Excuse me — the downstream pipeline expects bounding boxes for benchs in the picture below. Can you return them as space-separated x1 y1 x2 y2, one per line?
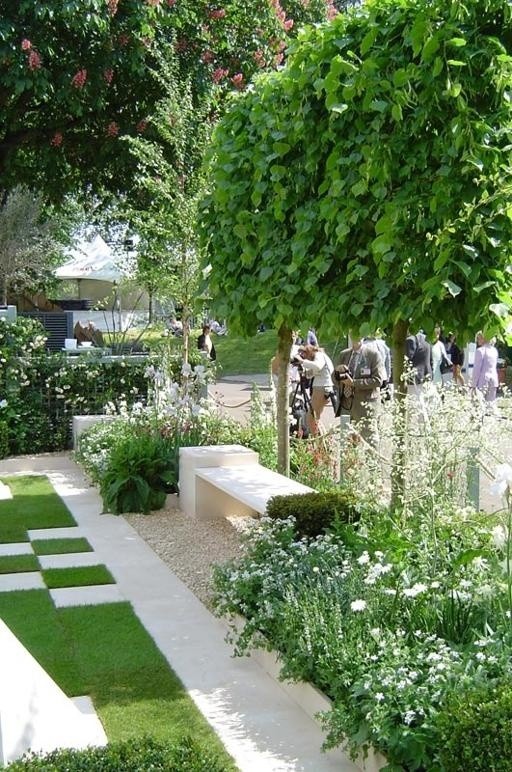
178 443 318 518
63 347 112 356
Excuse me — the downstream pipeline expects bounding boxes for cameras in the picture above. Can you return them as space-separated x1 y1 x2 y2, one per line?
290 357 303 368
335 366 352 381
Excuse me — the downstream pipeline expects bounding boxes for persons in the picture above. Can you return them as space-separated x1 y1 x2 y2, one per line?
161 315 216 363
271 319 499 477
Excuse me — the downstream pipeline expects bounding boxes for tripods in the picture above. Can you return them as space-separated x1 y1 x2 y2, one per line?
289 369 315 438
